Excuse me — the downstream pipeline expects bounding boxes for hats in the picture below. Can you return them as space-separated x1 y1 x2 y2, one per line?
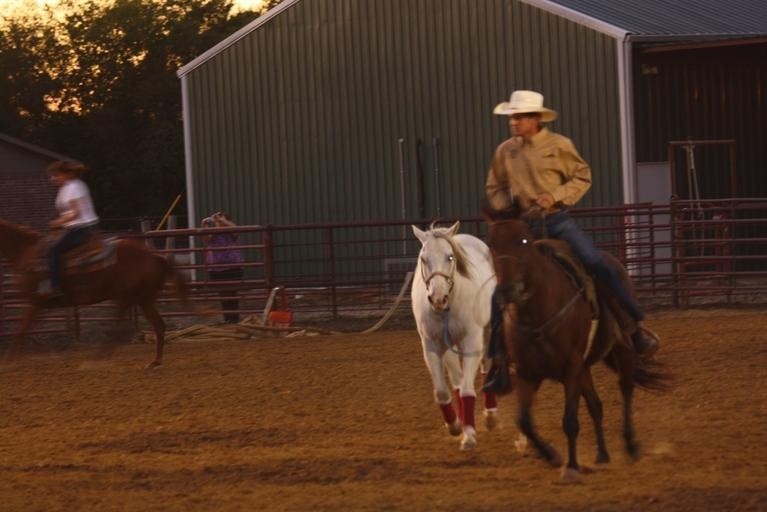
493 90 557 123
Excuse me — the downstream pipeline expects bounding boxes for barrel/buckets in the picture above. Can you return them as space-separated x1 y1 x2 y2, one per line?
268 312 292 335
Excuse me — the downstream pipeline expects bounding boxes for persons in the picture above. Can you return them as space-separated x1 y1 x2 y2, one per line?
481 86 679 399
200 212 246 329
34 156 103 301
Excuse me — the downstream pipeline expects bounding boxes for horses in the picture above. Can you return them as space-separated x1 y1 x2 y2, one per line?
0 219 197 371
410 220 498 457
479 194 674 482
